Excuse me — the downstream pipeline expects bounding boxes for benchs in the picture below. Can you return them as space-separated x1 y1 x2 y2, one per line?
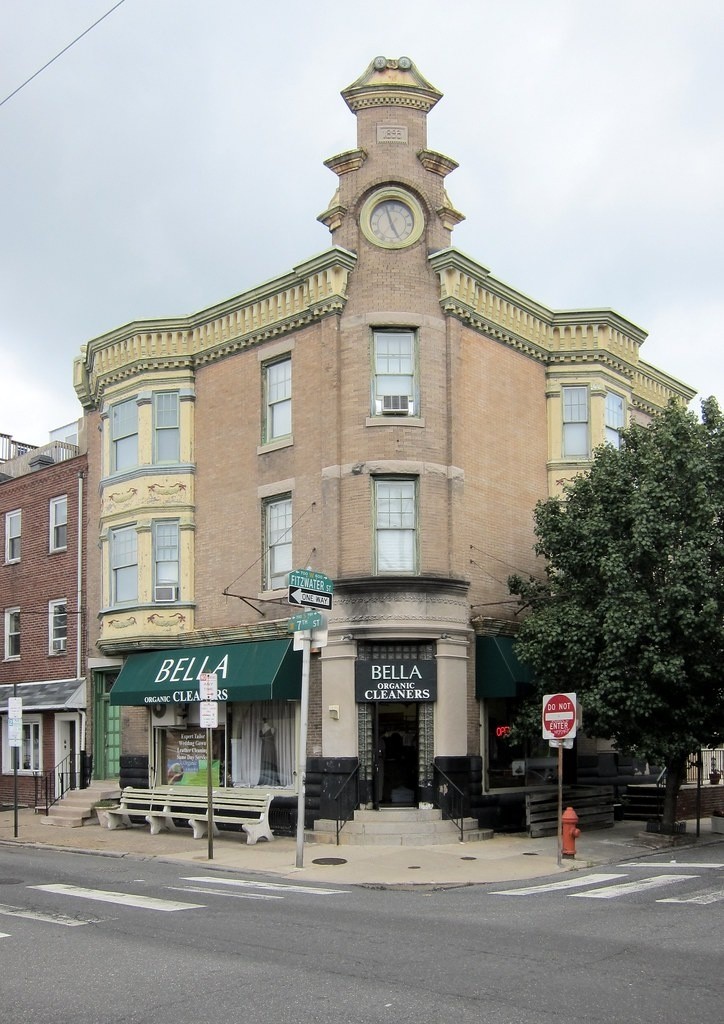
107 786 274 845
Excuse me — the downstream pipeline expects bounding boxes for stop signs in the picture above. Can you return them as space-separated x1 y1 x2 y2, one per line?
542 693 577 739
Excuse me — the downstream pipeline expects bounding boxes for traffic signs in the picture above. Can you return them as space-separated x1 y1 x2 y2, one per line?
287 585 333 612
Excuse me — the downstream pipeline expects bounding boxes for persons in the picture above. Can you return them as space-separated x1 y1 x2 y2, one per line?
257 717 280 786
167 763 184 785
377 727 387 804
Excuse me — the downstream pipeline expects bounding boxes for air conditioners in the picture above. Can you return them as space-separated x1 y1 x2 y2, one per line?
53 639 67 650
151 704 187 729
381 395 408 415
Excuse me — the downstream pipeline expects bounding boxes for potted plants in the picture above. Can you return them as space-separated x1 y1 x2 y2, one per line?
94 801 121 828
709 762 720 785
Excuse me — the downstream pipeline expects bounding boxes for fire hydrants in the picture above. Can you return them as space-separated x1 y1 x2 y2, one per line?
561 807 581 858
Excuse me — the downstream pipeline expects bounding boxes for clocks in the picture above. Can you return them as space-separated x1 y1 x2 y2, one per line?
358 187 425 249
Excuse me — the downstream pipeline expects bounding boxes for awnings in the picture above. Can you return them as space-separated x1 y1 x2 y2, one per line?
475 634 538 700
109 638 304 707
